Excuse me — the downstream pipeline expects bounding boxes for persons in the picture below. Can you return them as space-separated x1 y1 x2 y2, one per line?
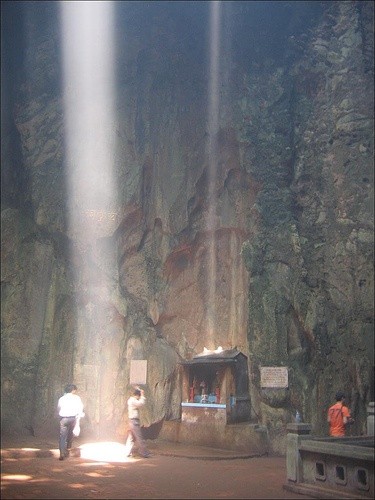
57 384 85 461
326 393 354 440
124 387 152 458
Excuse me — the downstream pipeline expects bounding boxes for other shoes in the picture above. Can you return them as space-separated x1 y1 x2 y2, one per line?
59 456 65 460
64 451 69 457
